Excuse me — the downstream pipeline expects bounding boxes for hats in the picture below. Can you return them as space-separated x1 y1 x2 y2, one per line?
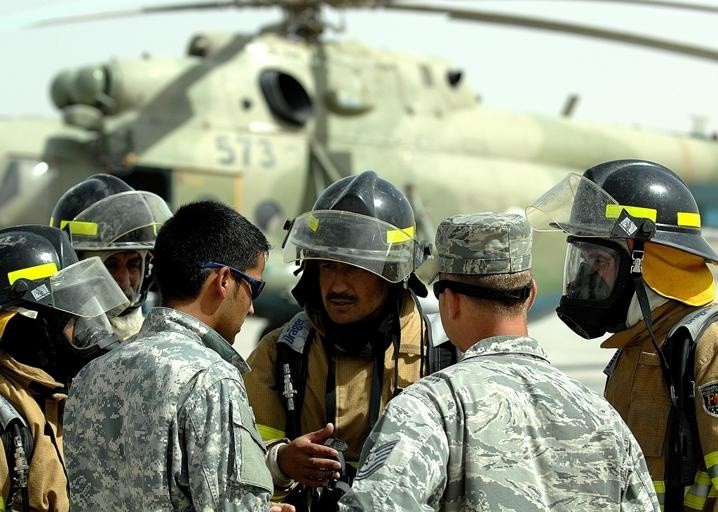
428 212 533 284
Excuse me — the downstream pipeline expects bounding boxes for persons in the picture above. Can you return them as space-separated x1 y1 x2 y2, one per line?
527 158 717 512
242 171 466 512
336 211 662 512
0 226 132 512
63 199 296 512
49 171 173 341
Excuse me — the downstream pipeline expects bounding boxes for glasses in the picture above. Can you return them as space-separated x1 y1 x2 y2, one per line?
198 258 267 300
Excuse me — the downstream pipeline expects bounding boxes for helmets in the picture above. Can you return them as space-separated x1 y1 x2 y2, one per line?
0 174 174 317
281 171 432 284
549 160 718 265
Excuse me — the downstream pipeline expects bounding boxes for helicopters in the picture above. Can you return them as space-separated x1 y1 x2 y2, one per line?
0 0 718 345
